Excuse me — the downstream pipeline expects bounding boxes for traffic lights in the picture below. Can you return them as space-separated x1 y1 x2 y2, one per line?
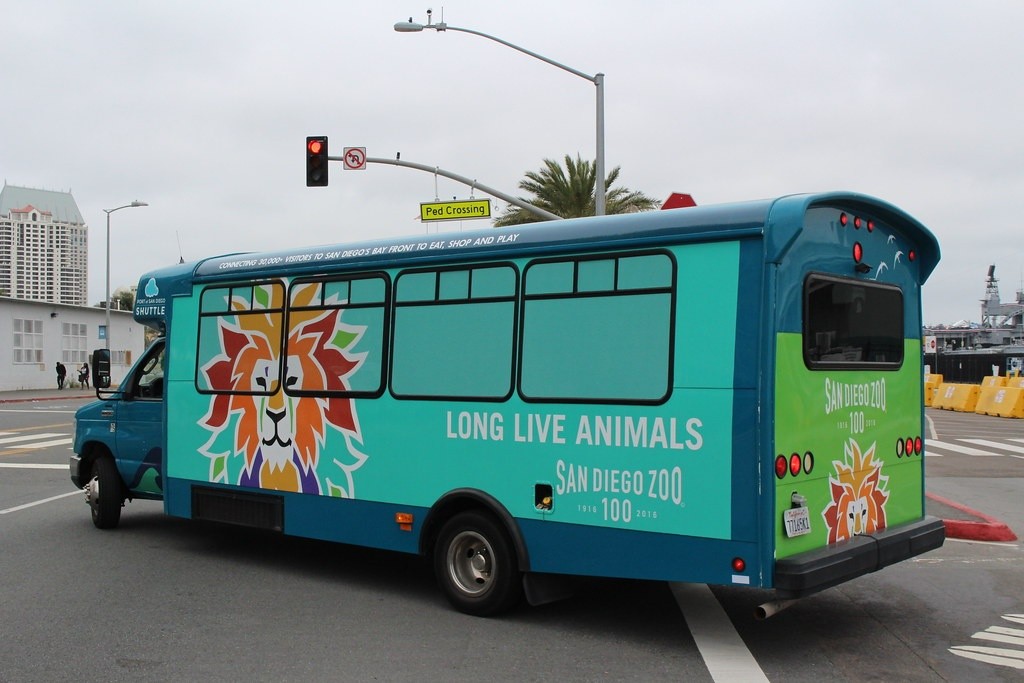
305 136 328 187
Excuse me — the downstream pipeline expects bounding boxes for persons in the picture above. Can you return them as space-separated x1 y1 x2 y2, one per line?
56 362 66 390
77 363 89 390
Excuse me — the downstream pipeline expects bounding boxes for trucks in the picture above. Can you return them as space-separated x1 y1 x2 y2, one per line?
69 190 948 619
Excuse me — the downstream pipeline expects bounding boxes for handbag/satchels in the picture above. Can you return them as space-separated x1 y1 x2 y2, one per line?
78 373 84 382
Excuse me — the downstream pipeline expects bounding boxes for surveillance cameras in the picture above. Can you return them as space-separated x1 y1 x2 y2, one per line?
427 8 432 15
397 152 400 160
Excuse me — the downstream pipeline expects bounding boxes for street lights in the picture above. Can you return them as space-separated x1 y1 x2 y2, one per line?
102 200 149 349
394 6 605 216
